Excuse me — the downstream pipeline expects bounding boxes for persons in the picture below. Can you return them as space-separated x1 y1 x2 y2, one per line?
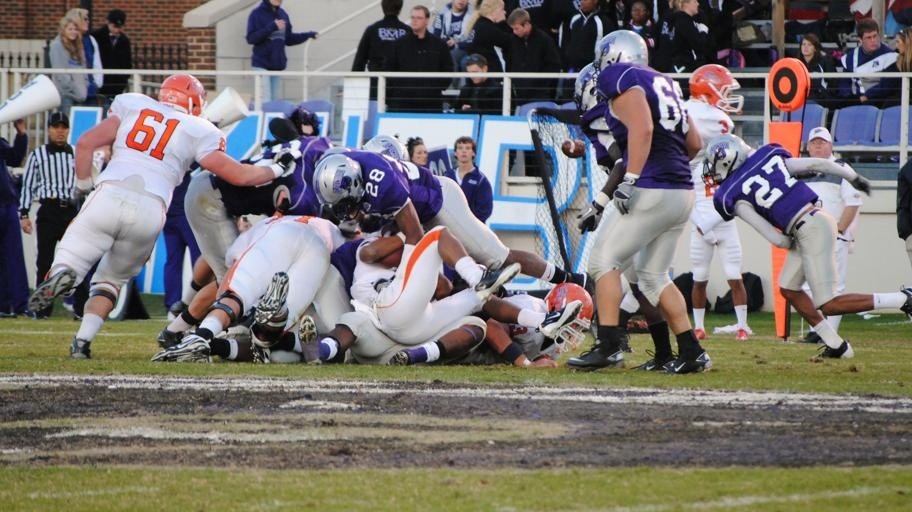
1 1 912 374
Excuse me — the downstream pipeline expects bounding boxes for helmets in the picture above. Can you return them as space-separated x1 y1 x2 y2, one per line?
573 29 747 189
157 74 410 220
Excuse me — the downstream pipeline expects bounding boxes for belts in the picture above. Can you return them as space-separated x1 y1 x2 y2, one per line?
796 209 819 229
60 200 68 208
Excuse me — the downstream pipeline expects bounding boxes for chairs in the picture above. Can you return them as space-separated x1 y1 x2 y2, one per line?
878 105 912 154
778 104 829 155
829 105 881 157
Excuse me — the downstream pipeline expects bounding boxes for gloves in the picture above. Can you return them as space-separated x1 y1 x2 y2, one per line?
613 172 641 215
852 176 871 195
577 200 604 234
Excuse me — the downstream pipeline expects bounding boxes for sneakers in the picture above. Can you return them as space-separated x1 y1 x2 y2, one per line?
900 288 912 316
811 340 854 362
69 335 91 359
797 331 823 344
26 269 76 313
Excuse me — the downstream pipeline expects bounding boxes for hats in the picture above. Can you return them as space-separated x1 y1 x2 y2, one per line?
48 111 69 128
809 126 831 142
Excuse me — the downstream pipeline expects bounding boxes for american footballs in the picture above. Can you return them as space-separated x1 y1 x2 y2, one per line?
561 140 586 158
